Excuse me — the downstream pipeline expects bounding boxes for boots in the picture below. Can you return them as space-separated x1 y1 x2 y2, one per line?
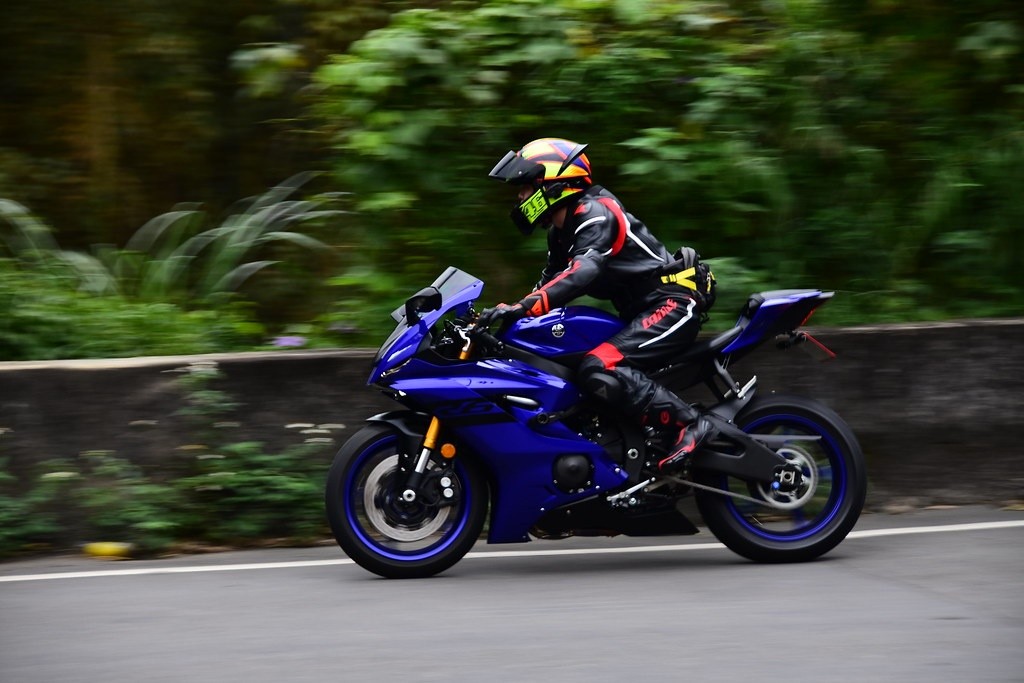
640 384 714 472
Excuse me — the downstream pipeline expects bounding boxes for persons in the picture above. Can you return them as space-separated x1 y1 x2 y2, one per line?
473 138 714 470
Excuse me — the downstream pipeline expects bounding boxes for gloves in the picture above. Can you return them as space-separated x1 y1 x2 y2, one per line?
477 302 526 337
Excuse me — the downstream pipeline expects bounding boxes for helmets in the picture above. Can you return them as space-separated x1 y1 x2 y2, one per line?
499 137 592 235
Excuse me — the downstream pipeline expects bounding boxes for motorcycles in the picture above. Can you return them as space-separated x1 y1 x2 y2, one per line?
326 265 867 579
